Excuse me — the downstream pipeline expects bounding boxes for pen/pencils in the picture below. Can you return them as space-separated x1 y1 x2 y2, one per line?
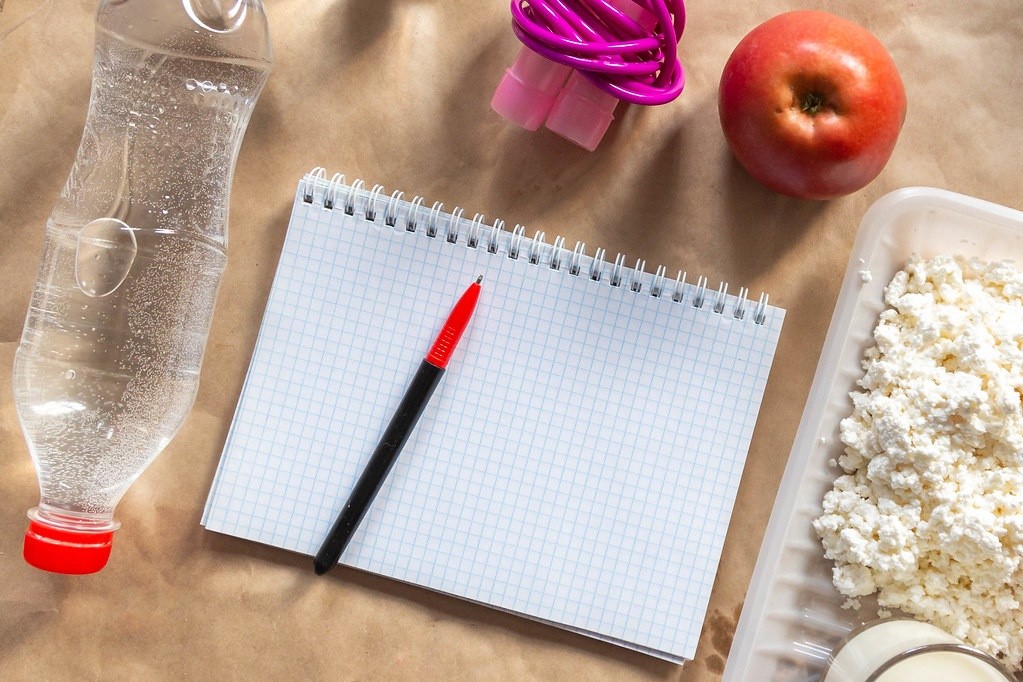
314 274 484 576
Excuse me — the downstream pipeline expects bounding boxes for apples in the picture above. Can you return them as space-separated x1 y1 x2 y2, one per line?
717 9 906 201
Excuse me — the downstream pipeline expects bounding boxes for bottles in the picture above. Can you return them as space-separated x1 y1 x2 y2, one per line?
10 0 273 576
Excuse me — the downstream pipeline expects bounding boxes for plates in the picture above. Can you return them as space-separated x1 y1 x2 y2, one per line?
719 185 1023 682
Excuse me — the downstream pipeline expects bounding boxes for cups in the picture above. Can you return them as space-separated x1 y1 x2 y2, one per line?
819 615 1019 682
